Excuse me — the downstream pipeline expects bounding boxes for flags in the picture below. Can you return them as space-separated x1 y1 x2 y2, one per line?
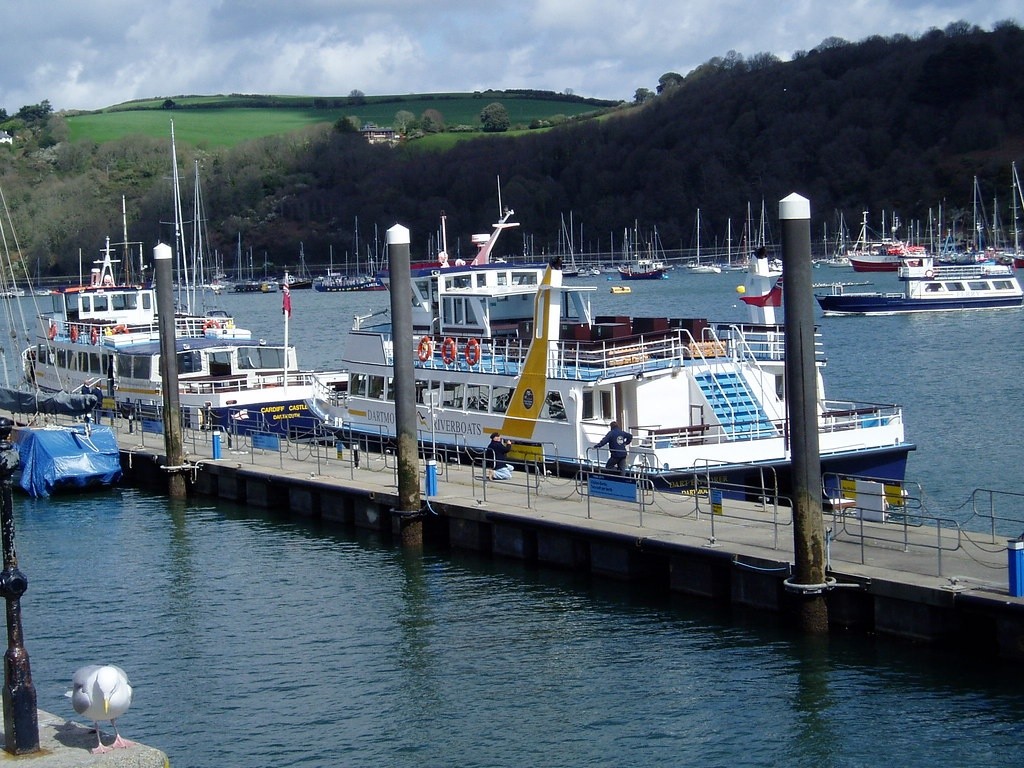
739 273 783 308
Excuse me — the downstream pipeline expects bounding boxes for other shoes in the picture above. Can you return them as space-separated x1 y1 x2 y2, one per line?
489 470 494 482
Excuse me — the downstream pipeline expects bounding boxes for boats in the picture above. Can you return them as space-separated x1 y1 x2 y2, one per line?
306 209 918 522
807 255 1024 318
19 236 351 435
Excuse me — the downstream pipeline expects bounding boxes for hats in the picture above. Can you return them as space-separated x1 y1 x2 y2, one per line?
490 432 499 438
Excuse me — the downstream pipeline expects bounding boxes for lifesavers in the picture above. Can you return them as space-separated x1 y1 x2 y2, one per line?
112 326 129 334
465 338 480 365
925 269 933 277
203 320 220 334
91 327 97 345
49 324 57 340
417 337 431 362
442 338 455 364
71 325 76 342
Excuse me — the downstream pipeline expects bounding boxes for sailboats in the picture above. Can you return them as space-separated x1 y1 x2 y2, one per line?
0 158 1023 299
0 145 120 496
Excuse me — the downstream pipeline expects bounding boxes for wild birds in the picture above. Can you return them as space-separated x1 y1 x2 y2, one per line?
65 664 135 754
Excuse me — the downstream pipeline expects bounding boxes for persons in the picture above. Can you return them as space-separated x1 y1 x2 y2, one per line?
592 421 632 483
486 432 514 480
